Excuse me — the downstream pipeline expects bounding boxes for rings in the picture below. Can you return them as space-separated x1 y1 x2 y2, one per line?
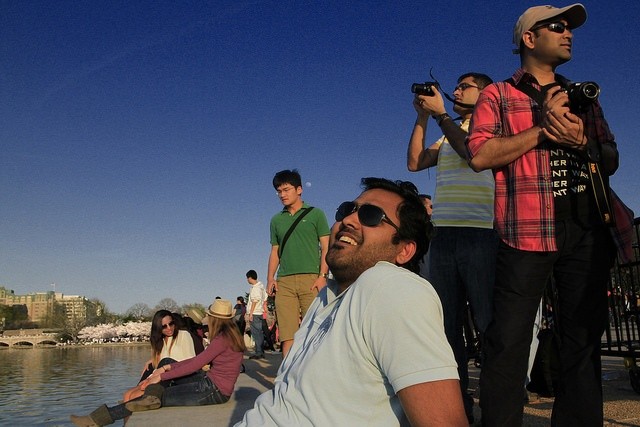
418 100 422 105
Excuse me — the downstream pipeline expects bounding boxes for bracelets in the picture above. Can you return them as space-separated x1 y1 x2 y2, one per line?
162 365 166 371
319 272 329 277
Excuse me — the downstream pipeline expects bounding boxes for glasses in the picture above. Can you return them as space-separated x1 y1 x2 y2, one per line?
455 83 478 91
162 321 174 329
335 201 399 232
424 205 432 209
275 185 294 196
531 21 573 34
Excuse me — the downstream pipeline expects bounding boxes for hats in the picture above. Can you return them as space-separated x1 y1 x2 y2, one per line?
512 3 588 54
204 299 237 319
184 308 204 325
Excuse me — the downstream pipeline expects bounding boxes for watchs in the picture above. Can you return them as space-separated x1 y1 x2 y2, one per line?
436 112 449 126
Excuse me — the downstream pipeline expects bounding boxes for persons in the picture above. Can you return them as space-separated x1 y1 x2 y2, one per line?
234 176 471 427
69 297 244 427
468 2 619 427
245 270 268 358
265 168 331 362
207 296 221 308
132 309 211 384
234 291 285 353
407 72 502 423
416 193 436 278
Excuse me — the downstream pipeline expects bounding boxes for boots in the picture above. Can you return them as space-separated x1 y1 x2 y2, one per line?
69 403 115 427
125 384 164 412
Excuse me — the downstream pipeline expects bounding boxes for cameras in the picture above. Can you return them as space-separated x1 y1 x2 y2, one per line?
551 81 601 115
411 81 438 96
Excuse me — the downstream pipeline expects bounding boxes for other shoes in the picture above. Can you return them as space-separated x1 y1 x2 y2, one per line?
249 354 265 359
